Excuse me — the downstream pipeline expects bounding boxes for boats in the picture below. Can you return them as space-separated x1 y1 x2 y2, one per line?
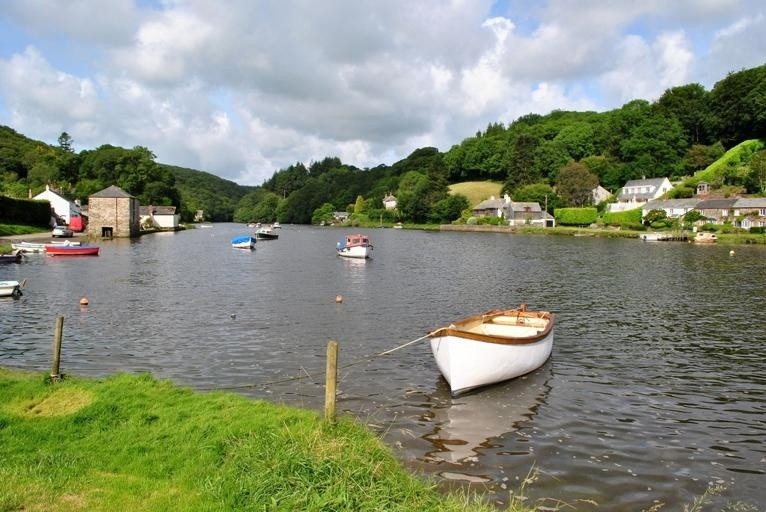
427 304 555 398
394 222 404 229
201 225 214 229
336 234 373 259
0 281 20 297
0 239 99 266
694 231 719 243
231 222 281 249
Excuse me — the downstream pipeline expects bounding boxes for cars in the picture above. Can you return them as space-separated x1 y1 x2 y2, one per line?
52 226 73 238
